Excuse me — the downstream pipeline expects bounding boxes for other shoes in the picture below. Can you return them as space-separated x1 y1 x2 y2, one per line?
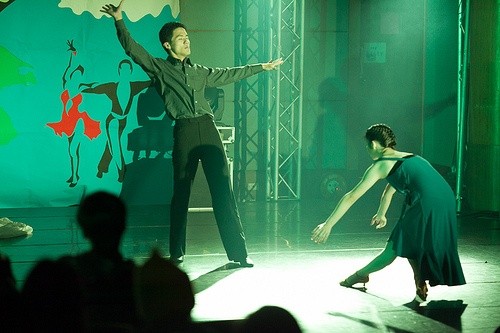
171 256 183 263
234 260 253 267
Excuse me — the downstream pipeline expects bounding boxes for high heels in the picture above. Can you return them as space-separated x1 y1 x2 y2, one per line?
414 274 428 297
340 272 369 286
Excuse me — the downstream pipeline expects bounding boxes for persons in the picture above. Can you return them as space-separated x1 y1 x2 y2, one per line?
309 123 466 297
100 0 283 268
0 192 302 333
